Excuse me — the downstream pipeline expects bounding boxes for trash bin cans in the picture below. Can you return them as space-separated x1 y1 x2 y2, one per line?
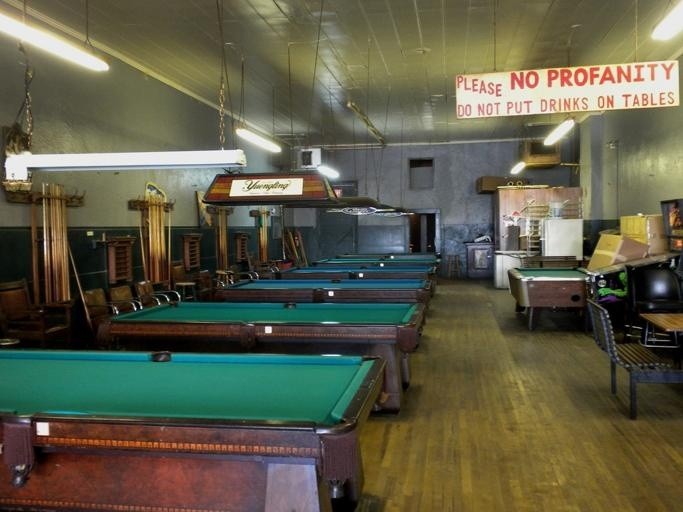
494 250 527 288
464 242 494 279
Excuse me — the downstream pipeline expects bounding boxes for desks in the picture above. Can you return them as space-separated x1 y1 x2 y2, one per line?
0 349 387 512
210 253 441 353
508 267 594 331
97 298 427 415
639 313 683 347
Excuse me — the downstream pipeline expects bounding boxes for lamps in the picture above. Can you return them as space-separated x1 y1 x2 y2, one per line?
0 0 112 72
235 55 282 154
316 90 341 180
0 0 248 170
542 114 575 146
508 128 527 175
651 0 683 39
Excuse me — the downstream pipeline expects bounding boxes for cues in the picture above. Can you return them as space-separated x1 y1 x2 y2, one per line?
29 181 71 304
66 241 92 323
257 205 269 261
137 191 171 291
215 205 229 270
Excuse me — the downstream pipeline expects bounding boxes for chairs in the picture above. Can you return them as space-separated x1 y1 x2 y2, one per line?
586 299 683 422
1 267 261 349
628 269 683 348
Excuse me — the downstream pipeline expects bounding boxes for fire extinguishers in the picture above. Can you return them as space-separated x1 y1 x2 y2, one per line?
294 228 300 247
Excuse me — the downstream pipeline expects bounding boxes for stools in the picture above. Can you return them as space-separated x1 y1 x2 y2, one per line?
447 254 462 280
